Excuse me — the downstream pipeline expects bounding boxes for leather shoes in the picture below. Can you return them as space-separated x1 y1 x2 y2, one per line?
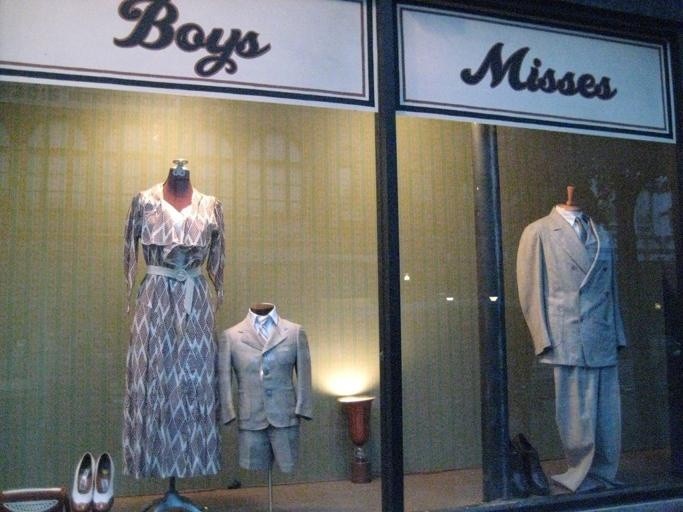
71 451 95 512
93 451 115 512
505 433 551 500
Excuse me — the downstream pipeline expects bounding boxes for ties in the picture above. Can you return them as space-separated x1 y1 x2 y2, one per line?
575 216 587 245
257 319 270 344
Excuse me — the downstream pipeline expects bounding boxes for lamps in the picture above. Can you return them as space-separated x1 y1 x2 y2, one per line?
337 395 380 484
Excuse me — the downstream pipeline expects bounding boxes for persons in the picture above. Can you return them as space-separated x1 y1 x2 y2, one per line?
119 159 226 479
516 185 627 497
218 302 314 474
